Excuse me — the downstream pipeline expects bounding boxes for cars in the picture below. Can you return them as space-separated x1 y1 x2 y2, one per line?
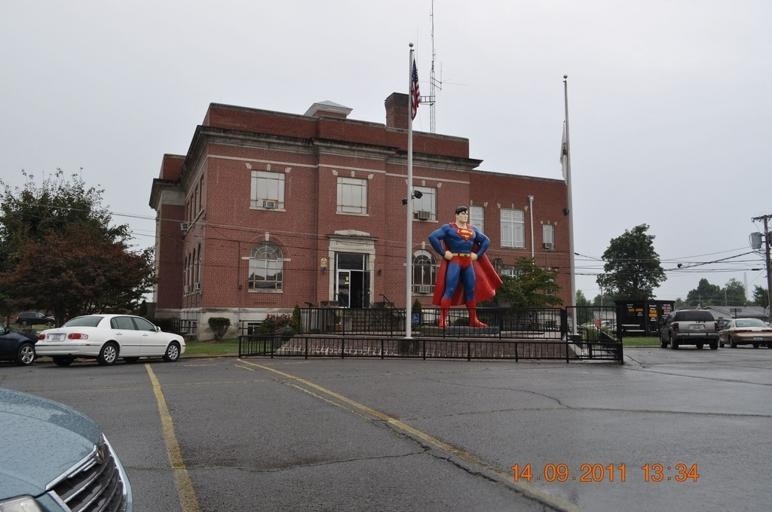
14 310 56 327
578 319 618 333
0 324 36 367
720 317 772 348
0 386 137 512
33 312 189 368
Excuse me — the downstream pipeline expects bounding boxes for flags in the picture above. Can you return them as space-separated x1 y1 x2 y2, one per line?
559 119 568 186
410 57 421 121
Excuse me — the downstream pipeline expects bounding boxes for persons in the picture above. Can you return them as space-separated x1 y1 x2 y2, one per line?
428 205 505 327
560 305 570 341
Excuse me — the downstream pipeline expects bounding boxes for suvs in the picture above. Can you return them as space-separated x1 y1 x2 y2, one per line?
661 310 720 351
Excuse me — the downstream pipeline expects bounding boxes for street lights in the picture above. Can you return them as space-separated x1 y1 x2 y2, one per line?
562 204 579 337
572 250 607 264
400 189 422 339
